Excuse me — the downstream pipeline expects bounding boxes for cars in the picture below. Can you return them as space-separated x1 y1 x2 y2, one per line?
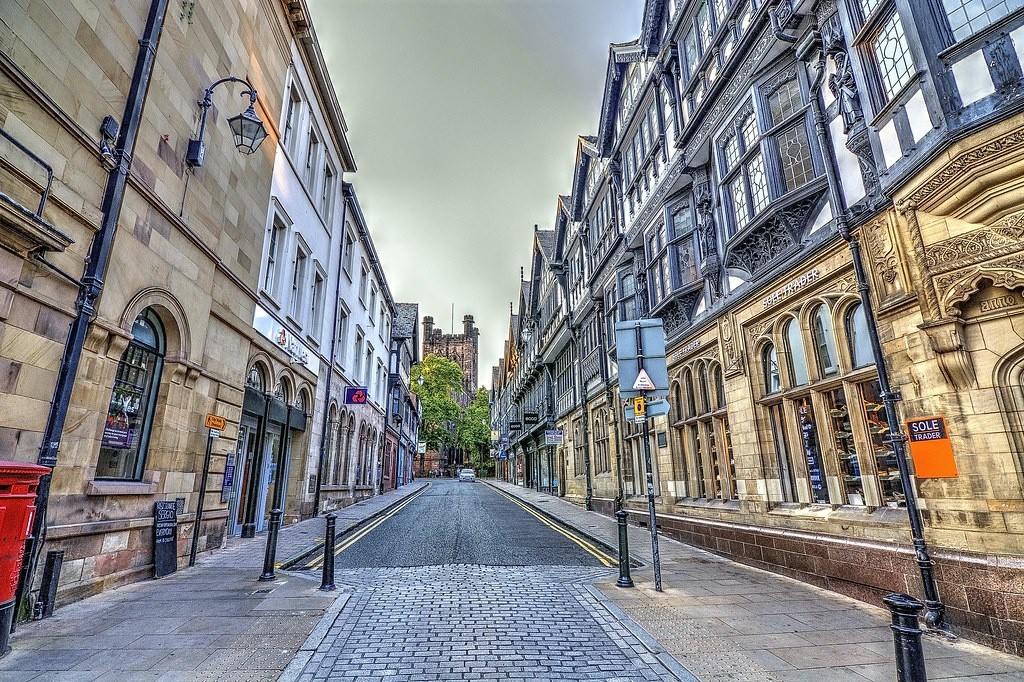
459 469 476 483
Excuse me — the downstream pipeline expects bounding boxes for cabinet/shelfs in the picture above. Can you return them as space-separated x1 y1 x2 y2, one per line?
100 406 139 453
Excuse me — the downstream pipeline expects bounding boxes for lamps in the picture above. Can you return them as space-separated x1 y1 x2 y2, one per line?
188 75 271 167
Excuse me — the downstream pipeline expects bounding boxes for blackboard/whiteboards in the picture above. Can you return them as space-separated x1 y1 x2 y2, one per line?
152 500 178 579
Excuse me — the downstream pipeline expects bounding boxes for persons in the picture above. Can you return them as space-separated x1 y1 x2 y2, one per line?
472 467 478 476
457 468 460 477
432 468 444 479
